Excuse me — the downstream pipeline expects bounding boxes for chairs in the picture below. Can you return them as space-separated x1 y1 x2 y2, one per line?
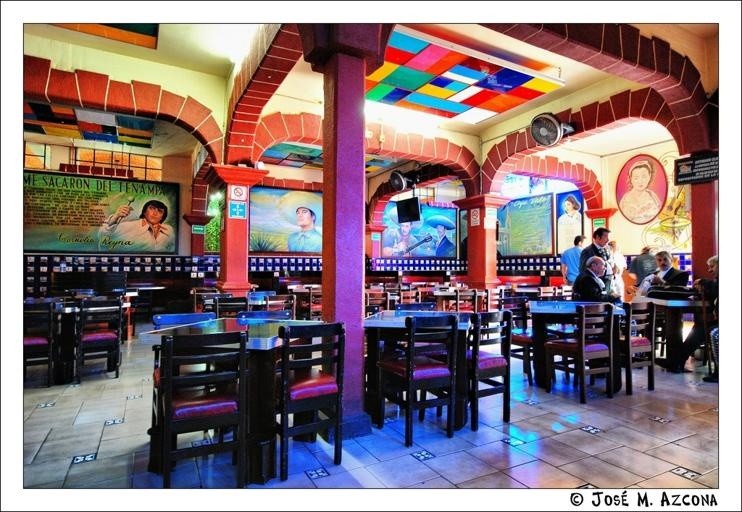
160 331 249 490
202 293 232 311
297 287 322 320
701 285 719 382
365 292 390 315
274 320 345 480
265 294 296 320
620 302 657 395
191 287 219 313
562 285 574 301
455 288 477 318
466 310 513 431
375 316 458 448
247 290 276 301
544 303 614 403
498 296 556 387
213 296 249 318
400 291 422 304
537 286 557 300
75 298 123 383
236 310 291 319
484 288 502 344
153 313 216 330
654 321 667 357
23 301 54 387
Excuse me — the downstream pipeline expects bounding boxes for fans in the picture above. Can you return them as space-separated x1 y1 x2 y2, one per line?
390 170 419 192
527 111 577 147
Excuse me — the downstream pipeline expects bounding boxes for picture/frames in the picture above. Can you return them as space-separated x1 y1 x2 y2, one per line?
497 193 557 256
203 184 325 255
556 189 584 257
24 169 181 255
380 200 461 260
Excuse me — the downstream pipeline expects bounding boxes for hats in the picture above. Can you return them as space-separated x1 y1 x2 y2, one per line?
285 201 322 227
390 206 425 230
427 215 456 231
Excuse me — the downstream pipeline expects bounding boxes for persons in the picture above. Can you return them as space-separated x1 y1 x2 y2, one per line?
572 256 622 302
608 241 627 302
426 215 456 257
645 251 689 343
383 206 423 258
578 228 613 292
560 235 586 286
275 191 323 253
618 160 662 224
558 195 582 225
654 256 719 371
98 200 174 252
630 246 656 287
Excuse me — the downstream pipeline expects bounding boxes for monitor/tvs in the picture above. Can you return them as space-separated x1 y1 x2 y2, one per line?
396 196 420 223
